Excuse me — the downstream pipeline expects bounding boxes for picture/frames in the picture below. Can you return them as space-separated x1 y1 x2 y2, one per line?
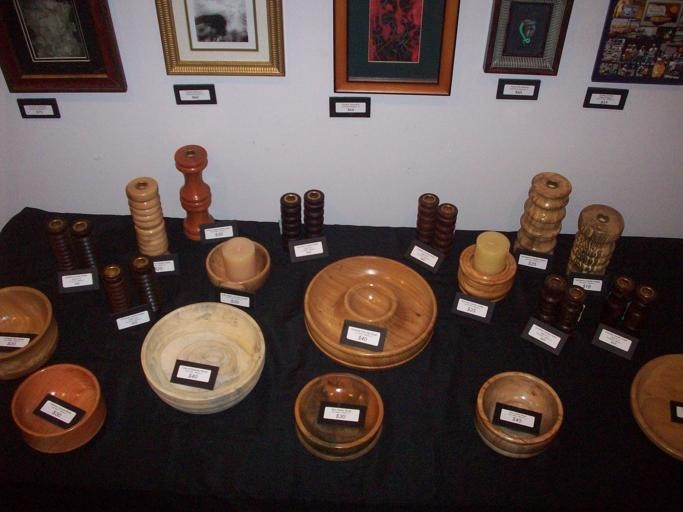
0 1 127 94
483 0 573 76
591 0 682 86
155 1 285 77
331 0 460 97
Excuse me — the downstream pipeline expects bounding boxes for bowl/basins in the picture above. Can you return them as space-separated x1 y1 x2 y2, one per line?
11 364 108 456
470 371 567 459
0 283 59 378
137 300 268 416
203 238 272 292
292 373 391 458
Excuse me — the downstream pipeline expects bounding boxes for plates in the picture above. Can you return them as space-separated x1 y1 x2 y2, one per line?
629 350 683 462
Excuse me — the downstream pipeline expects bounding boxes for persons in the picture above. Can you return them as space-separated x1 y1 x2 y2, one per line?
599 40 683 81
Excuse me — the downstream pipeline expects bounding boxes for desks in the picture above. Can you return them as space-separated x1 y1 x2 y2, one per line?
0 206 683 511
